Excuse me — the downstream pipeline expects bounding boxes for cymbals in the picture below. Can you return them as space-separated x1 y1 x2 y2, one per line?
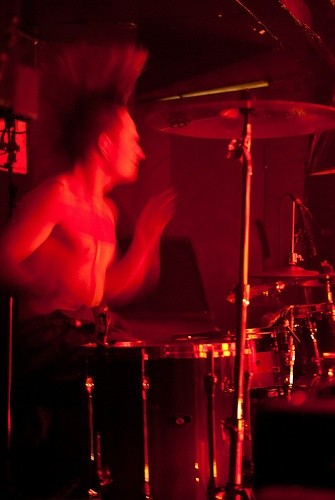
249 268 320 279
146 98 335 140
226 284 303 308
309 165 335 176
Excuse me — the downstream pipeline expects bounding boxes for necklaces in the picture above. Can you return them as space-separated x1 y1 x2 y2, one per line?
76 188 108 228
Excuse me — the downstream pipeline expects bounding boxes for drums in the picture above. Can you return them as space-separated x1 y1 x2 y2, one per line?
78 332 255 500
173 326 288 391
273 302 335 389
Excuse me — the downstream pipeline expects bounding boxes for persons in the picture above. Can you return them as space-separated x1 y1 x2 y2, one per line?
0 44 175 471
